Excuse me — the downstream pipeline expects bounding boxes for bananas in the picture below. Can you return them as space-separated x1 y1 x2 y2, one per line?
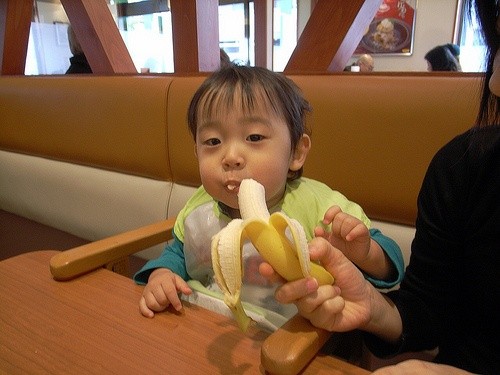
211 178 335 333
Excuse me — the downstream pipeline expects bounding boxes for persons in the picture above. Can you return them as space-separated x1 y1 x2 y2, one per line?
64 23 93 75
259 0 500 375
134 59 404 319
424 45 462 71
220 47 230 62
445 43 460 63
344 54 374 72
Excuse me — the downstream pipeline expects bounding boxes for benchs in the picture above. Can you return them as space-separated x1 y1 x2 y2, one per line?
0 72 500 337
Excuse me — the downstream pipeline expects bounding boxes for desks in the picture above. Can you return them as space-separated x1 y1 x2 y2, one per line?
0 250 375 375
